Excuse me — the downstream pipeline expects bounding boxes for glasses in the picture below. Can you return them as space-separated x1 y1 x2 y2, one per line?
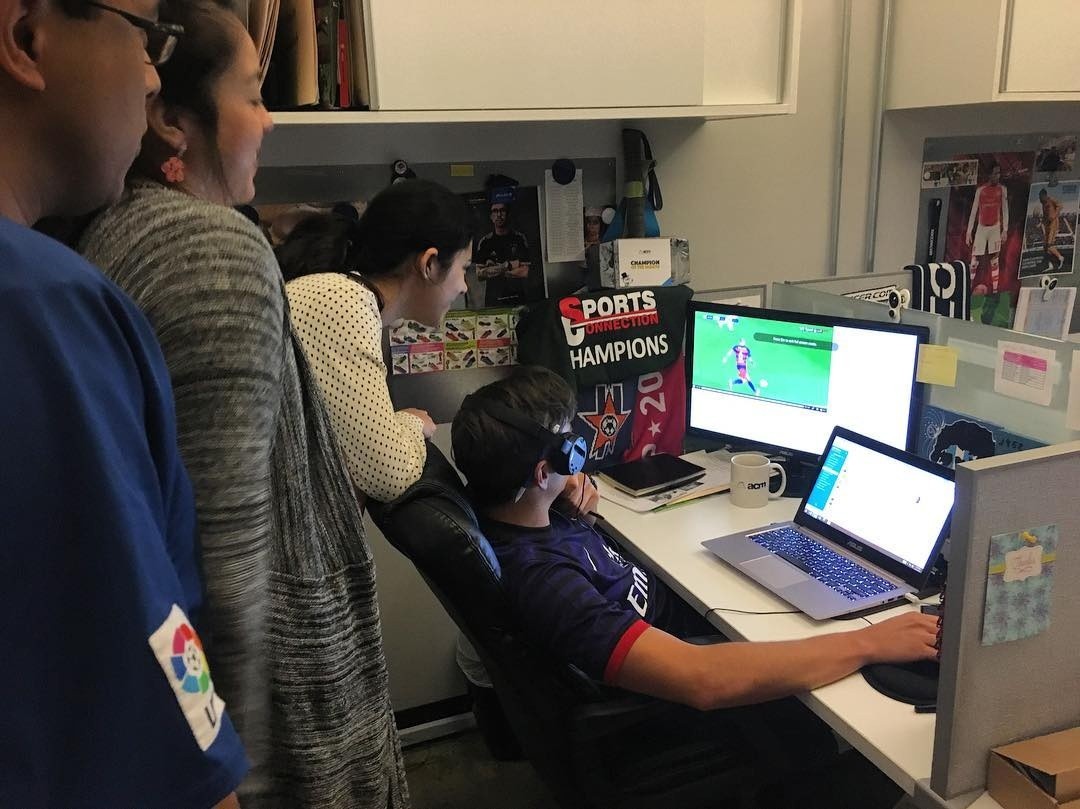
491 208 505 214
89 0 185 66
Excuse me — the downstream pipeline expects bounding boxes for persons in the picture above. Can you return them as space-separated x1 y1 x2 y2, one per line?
271 178 473 516
60 0 411 809
1038 189 1064 273
965 159 1008 293
0 0 252 809
1041 145 1074 170
722 337 760 396
451 365 944 809
476 197 531 306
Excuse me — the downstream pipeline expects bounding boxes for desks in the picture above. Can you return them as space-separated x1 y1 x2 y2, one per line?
590 452 936 797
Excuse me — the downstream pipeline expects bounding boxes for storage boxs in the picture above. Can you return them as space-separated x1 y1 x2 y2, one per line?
600 237 690 288
984 724 1080 809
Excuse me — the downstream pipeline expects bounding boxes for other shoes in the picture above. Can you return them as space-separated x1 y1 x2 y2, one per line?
1058 257 1064 270
1041 267 1054 274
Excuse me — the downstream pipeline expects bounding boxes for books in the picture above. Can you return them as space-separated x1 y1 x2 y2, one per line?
314 0 353 111
453 185 549 310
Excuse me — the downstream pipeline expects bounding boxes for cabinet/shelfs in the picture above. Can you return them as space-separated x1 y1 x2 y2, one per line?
231 0 802 123
890 0 1080 106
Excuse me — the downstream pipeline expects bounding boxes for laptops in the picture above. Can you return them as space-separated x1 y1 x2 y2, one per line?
698 424 957 621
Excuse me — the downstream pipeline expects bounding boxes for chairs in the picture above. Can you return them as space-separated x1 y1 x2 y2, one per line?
366 439 845 809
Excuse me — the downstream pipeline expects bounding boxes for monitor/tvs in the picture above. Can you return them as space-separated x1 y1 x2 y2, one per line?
683 299 929 498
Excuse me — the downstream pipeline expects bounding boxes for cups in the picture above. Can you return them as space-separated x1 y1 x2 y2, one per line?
730 454 787 508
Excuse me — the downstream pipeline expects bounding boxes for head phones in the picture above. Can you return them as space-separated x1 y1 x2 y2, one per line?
460 393 589 477
